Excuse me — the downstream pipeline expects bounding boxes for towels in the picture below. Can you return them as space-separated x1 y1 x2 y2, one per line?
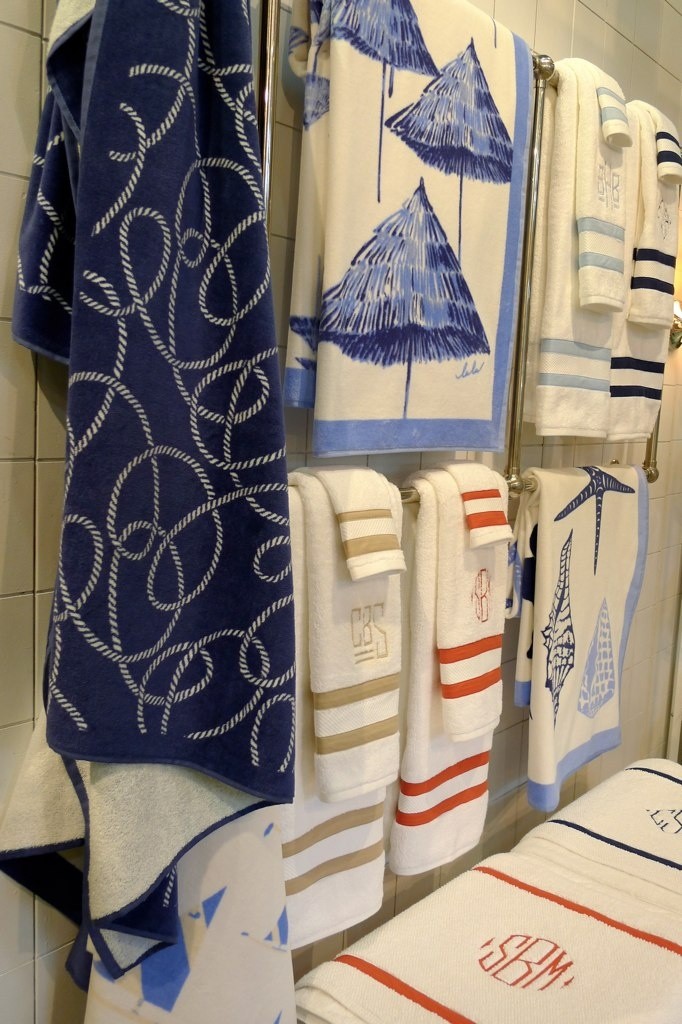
8 0 680 1024
